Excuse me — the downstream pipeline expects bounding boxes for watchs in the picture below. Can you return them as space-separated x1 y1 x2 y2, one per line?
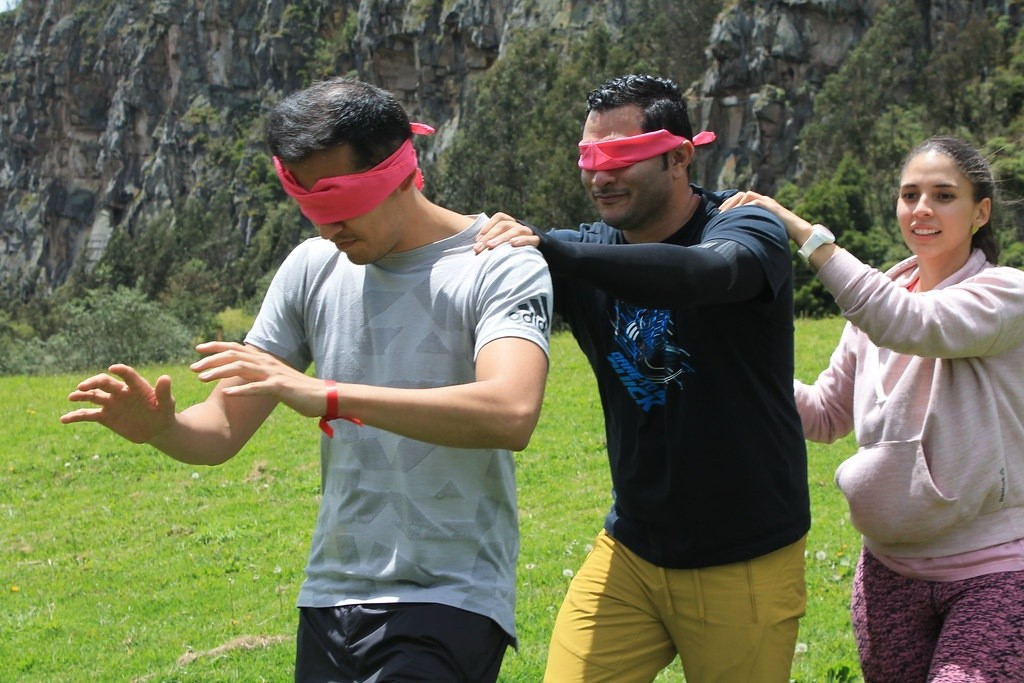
796 224 835 263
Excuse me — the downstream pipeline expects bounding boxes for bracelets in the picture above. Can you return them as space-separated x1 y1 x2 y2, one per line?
319 380 364 437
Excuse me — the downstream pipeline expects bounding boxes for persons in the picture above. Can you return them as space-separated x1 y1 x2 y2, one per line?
470 76 810 683
61 79 552 683
718 137 1023 683
